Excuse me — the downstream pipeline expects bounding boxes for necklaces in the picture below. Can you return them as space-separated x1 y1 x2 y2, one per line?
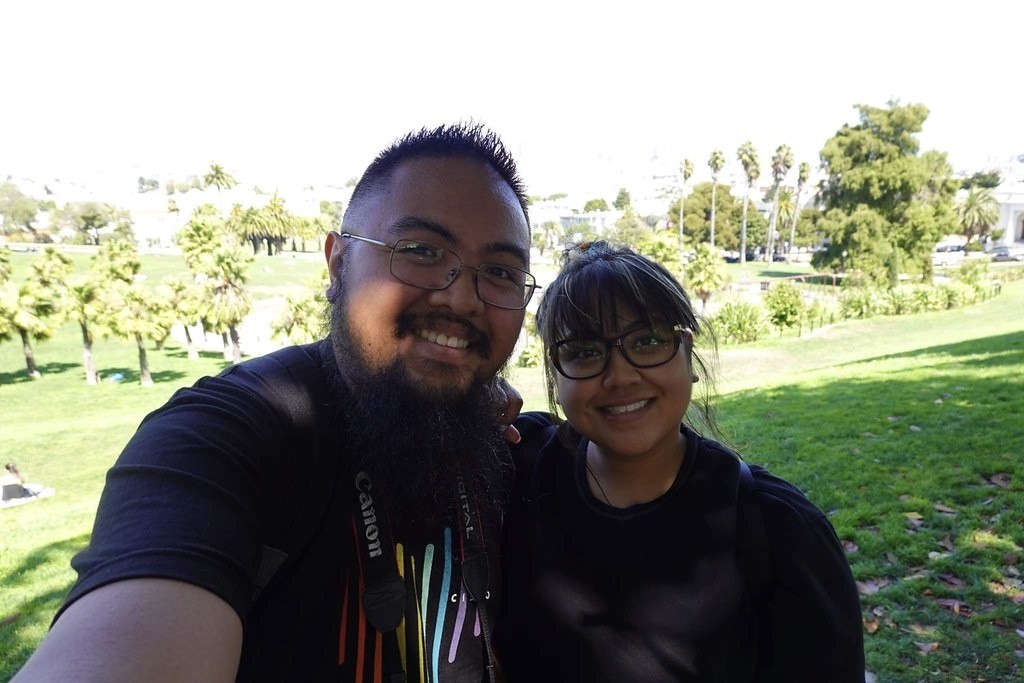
587 465 610 505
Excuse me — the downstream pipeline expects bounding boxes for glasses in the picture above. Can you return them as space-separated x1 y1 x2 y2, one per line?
548 318 681 380
342 232 541 311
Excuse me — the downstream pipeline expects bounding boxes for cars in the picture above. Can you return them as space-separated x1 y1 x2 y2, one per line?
987 244 1024 262
929 244 964 268
723 253 785 263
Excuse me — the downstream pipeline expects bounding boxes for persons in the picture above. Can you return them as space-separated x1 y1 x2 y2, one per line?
496 242 868 683
0 122 543 683
